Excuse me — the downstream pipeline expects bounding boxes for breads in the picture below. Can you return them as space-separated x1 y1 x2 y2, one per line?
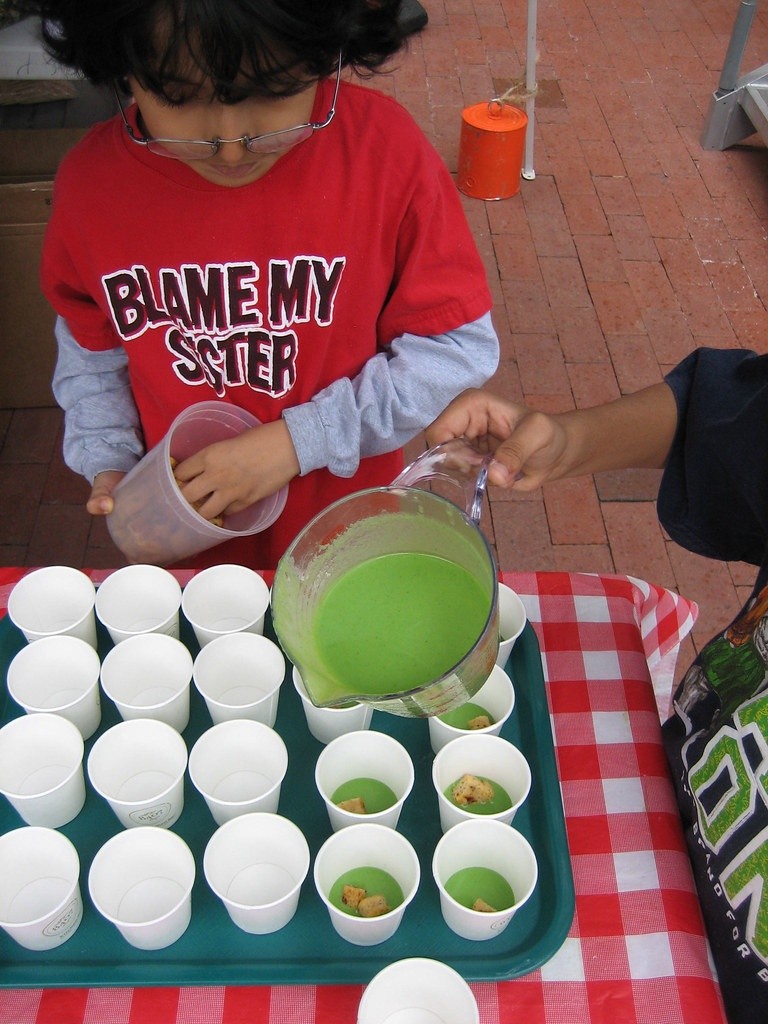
105 456 225 566
341 886 391 918
469 715 490 730
473 898 496 913
335 797 366 814
452 775 494 805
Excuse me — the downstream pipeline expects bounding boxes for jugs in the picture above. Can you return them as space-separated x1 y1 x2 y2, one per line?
268 436 499 718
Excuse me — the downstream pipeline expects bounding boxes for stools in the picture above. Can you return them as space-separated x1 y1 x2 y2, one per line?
1 0 129 130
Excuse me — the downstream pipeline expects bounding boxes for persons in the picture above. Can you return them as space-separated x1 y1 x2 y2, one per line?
423 349 768 1024
36 0 501 569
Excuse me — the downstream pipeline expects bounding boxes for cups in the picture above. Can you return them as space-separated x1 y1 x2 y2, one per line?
8 565 97 655
88 826 197 950
182 564 269 643
432 819 538 940
7 635 101 741
357 958 480 1024
291 583 533 834
100 633 191 734
192 632 285 729
0 712 85 829
313 825 420 946
87 718 188 829
0 826 84 951
189 719 289 826
96 564 182 645
204 810 309 936
105 401 289 563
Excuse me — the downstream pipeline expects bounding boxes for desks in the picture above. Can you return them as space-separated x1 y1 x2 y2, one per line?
0 568 729 1023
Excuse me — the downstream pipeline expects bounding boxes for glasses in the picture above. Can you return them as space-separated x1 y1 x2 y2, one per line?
105 39 343 161
